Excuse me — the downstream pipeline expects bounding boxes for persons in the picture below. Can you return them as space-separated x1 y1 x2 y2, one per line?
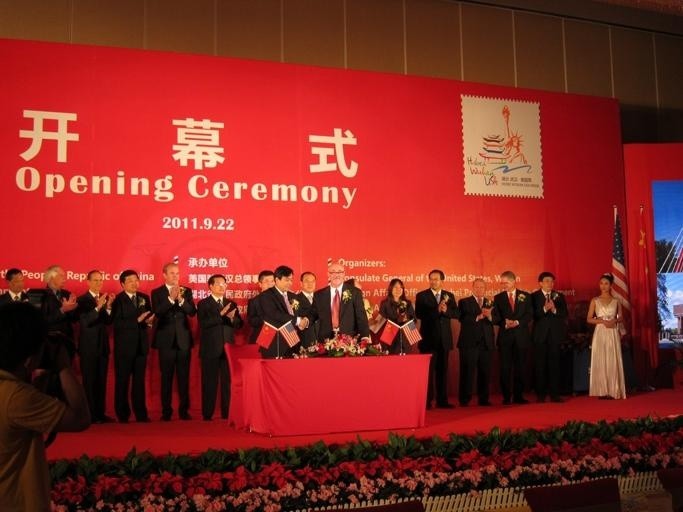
246 269 277 359
151 263 197 419
529 272 570 403
297 264 370 350
2 265 115 420
1 300 93 512
198 274 242 418
112 269 155 420
254 266 304 360
491 269 533 403
587 273 625 398
345 278 370 322
457 278 496 406
378 278 417 353
415 270 457 408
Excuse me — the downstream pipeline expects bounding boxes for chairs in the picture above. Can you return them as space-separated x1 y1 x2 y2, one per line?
225 343 261 431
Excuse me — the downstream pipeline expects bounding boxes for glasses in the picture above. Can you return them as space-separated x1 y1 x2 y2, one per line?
329 271 344 274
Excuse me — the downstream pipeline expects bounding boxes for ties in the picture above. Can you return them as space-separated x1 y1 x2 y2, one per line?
435 293 440 303
545 295 550 303
285 295 292 318
332 289 340 328
509 293 515 311
217 299 222 309
15 296 18 301
131 295 136 306
478 298 482 308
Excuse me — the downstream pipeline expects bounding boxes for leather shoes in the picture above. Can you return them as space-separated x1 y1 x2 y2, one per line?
606 396 614 400
599 396 606 400
426 396 565 408
90 414 229 423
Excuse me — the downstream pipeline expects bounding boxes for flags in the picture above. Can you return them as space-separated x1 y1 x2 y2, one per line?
403 321 421 343
610 205 631 347
256 321 276 348
628 204 660 369
380 321 398 345
279 322 300 347
